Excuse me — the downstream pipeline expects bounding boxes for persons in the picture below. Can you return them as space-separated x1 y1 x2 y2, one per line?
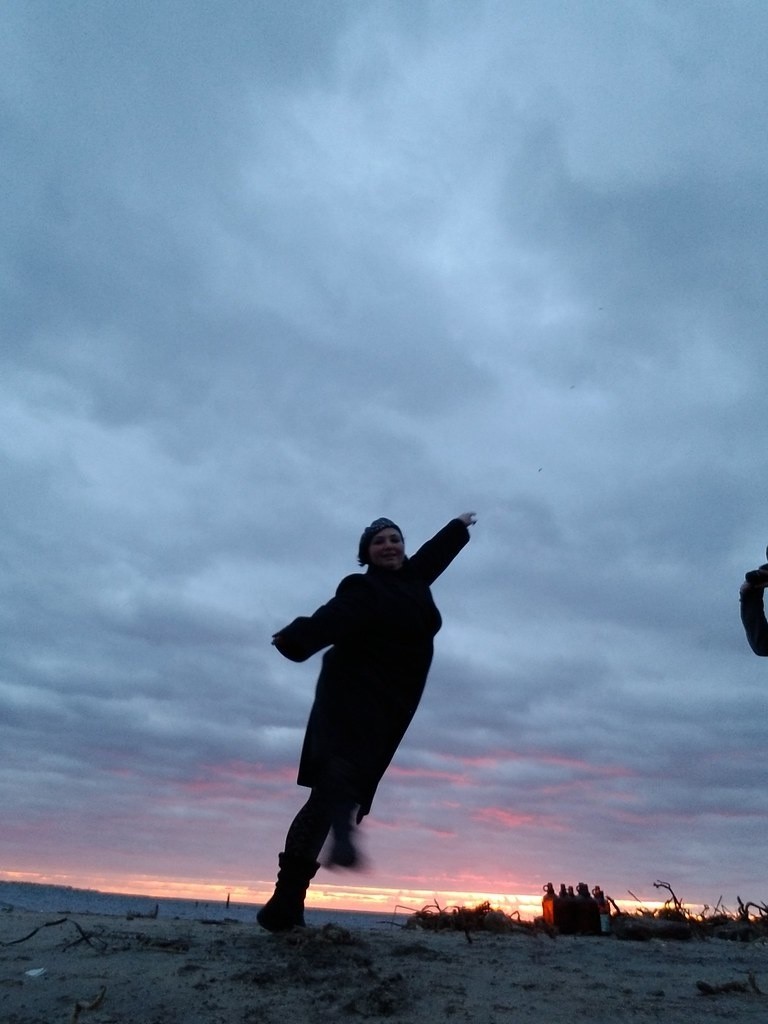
255 512 477 931
738 547 768 656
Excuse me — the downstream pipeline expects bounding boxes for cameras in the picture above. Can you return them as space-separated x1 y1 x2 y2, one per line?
745 569 768 586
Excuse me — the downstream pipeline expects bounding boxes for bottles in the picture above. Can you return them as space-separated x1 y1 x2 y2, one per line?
542 882 612 936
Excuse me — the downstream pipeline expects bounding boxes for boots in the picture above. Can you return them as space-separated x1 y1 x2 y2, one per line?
330 824 356 867
257 852 321 933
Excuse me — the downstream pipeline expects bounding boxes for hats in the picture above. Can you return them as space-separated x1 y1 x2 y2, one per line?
359 517 396 568
